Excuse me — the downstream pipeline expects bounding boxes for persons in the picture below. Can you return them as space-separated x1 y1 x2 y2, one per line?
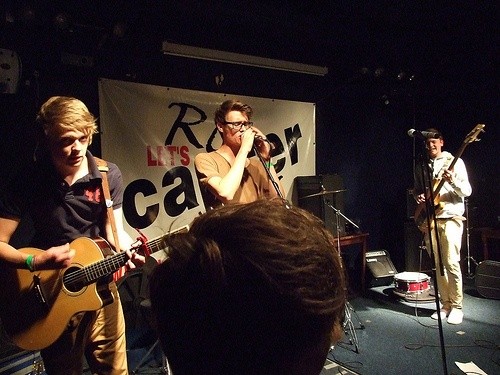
144 196 348 375
412 129 472 323
194 100 285 207
0 96 147 375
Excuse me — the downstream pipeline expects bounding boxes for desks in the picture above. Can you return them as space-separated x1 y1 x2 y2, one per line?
479 228 500 262
333 232 371 277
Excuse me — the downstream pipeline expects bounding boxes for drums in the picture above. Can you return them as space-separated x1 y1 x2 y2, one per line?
393 272 431 294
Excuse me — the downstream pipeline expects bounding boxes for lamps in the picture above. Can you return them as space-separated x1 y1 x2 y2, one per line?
362 62 421 107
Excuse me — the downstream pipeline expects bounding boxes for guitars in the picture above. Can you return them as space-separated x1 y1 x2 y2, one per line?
414 123 486 232
0 210 203 352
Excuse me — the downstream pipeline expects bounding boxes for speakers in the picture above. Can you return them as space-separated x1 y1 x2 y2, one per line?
365 250 398 288
328 233 369 299
296 174 345 238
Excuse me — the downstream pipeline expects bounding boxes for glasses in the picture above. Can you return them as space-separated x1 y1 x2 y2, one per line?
227 121 253 131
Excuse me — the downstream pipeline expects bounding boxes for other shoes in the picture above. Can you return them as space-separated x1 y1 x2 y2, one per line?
431 310 449 320
447 307 463 325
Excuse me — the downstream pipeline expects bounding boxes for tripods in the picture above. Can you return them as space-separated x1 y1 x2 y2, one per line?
323 200 366 354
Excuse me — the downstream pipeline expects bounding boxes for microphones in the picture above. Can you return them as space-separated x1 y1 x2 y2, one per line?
254 135 262 140
408 128 440 139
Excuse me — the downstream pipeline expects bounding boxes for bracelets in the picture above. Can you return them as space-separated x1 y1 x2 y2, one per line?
449 180 455 184
265 162 274 167
25 254 36 272
